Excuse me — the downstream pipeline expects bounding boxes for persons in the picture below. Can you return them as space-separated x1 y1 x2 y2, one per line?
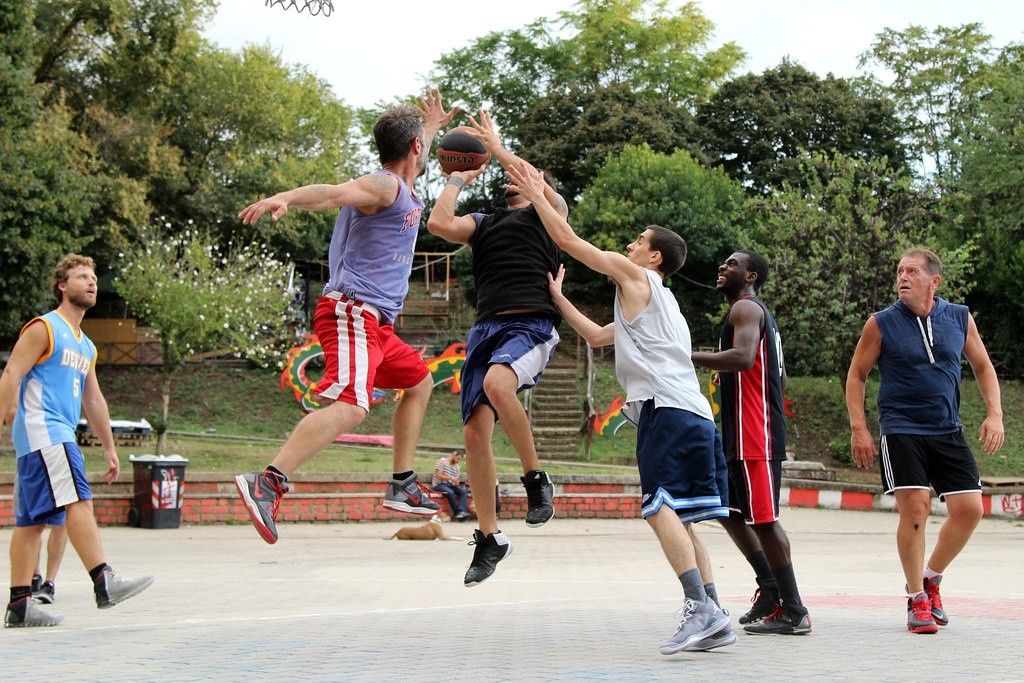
432 450 470 518
427 103 568 586
846 245 1004 634
235 88 443 546
504 162 739 656
0 252 154 628
692 251 814 635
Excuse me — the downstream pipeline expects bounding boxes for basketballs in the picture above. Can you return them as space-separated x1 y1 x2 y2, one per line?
436 125 490 173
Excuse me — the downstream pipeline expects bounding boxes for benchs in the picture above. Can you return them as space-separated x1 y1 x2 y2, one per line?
394 300 449 330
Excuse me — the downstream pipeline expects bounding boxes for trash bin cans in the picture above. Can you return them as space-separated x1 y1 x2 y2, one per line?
127 460 189 529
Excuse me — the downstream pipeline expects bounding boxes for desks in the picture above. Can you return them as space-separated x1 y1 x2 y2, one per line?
77 419 151 447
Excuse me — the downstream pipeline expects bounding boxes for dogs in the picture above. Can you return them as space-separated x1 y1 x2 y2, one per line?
384 512 465 541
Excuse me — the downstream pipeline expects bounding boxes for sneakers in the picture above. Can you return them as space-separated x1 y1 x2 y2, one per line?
37 581 53 602
5 592 63 628
683 608 736 651
521 470 555 527
31 576 42 596
907 592 939 633
382 473 442 515
660 594 731 655
924 575 948 626
464 529 514 587
234 469 289 544
743 600 811 635
739 588 778 624
94 566 153 609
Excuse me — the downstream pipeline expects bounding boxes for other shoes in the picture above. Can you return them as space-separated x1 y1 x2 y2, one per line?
456 512 470 521
